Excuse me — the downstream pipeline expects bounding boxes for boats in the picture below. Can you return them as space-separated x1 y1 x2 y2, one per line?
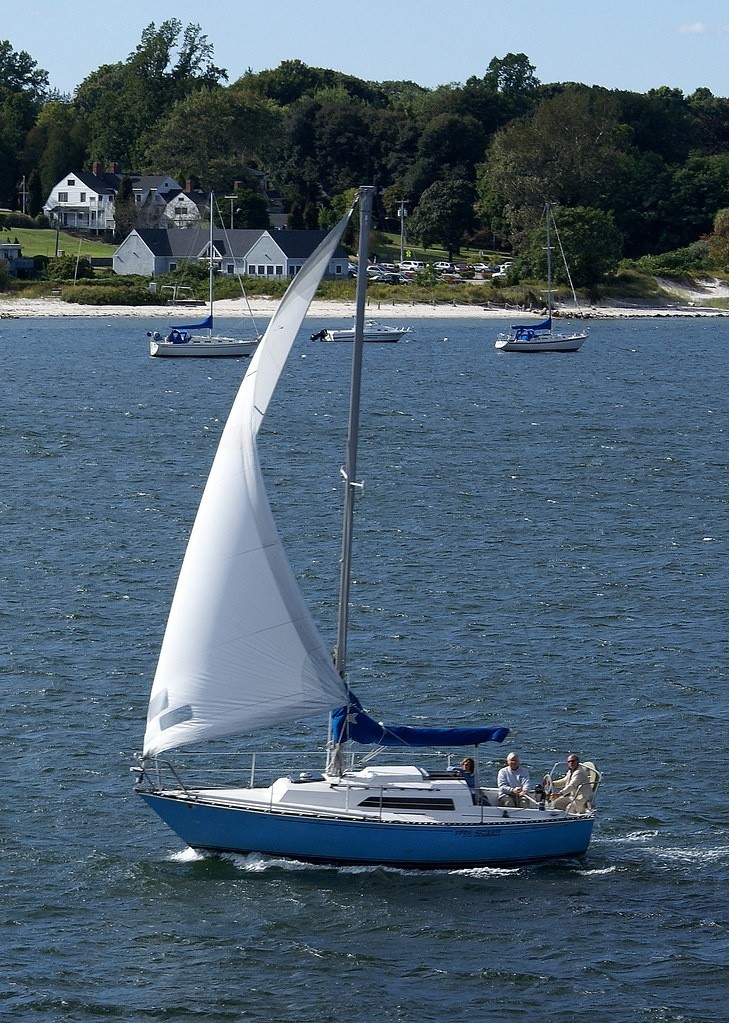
309 319 412 343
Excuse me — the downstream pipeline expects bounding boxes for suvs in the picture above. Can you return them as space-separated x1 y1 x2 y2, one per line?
345 260 516 287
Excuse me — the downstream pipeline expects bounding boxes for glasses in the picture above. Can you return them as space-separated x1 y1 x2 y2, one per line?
567 760 577 763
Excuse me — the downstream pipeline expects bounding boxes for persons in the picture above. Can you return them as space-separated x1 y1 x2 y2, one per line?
496 752 531 808
451 757 492 806
544 754 594 814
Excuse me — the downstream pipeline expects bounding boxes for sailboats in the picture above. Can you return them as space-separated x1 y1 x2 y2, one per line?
494 200 590 352
131 179 604 867
147 187 262 359
